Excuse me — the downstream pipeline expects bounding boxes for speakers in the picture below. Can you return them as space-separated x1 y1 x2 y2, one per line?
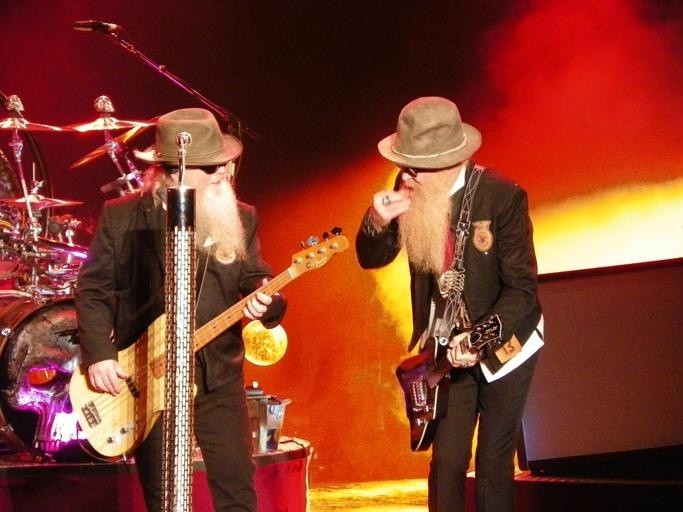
517 257 682 472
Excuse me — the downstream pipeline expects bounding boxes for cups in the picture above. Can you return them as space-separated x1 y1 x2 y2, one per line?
259 397 291 452
245 388 269 453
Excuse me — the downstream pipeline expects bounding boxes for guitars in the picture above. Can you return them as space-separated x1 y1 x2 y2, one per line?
397 315 502 451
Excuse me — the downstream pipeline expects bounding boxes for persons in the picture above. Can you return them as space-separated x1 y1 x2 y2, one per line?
75 108 288 512
355 94 545 512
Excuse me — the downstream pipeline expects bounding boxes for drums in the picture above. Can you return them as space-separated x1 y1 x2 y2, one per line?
0 295 84 457
0 253 21 292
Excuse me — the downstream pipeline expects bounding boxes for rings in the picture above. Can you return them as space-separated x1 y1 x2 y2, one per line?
461 362 469 369
382 195 390 205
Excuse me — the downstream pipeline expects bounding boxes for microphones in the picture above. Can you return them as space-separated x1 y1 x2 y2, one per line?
176 132 193 150
72 20 116 32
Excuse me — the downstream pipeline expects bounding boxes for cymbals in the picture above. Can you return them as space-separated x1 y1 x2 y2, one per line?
68 126 143 170
0 119 66 133
62 117 153 131
0 193 85 209
68 227 349 466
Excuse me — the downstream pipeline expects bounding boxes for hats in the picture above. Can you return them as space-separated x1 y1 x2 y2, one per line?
135 108 243 168
377 96 482 170
